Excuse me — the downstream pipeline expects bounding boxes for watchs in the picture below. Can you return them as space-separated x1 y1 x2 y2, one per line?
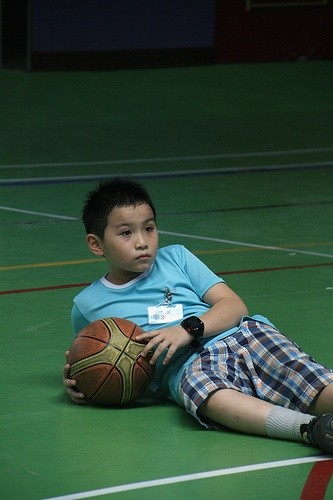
180 316 204 354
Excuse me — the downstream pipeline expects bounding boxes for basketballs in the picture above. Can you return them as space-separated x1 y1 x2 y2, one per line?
67 316 158 410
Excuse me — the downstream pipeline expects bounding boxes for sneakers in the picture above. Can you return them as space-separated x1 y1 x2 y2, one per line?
299 414 333 453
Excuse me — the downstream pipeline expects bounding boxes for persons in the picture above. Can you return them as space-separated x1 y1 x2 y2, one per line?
63 177 333 453
